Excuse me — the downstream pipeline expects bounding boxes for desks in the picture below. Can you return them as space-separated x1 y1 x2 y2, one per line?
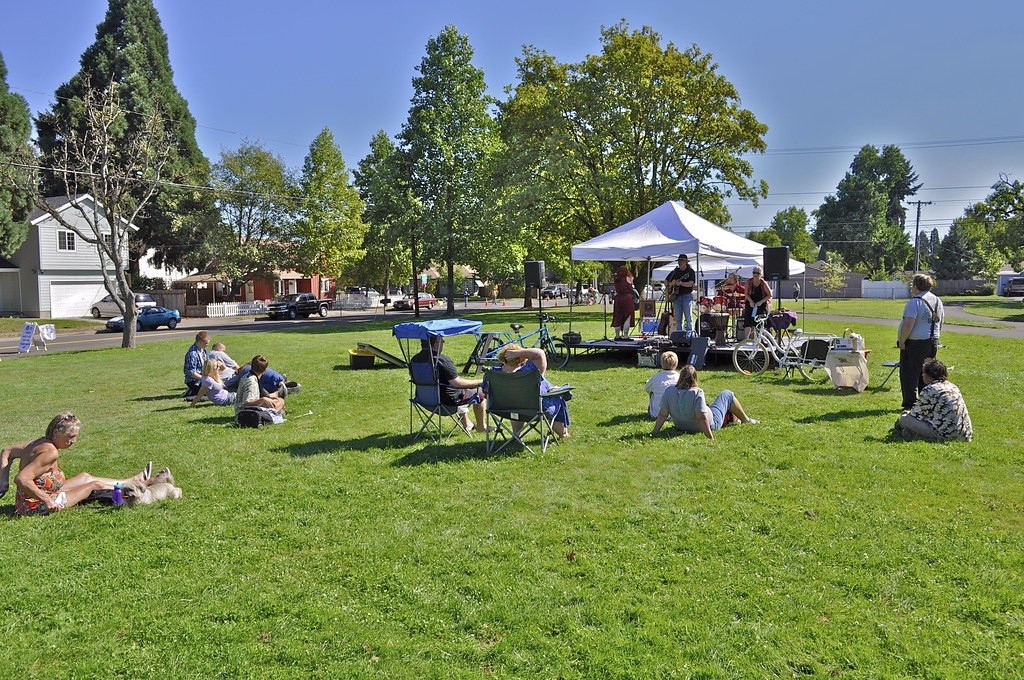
768 311 797 344
826 349 871 392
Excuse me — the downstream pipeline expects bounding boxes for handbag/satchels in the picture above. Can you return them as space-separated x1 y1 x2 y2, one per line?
237 406 281 428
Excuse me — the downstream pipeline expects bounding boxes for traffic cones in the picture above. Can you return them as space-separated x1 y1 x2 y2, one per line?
485 297 489 309
502 298 506 307
554 302 559 307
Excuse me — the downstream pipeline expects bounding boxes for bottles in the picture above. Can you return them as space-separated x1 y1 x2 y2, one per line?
112 483 123 506
752 306 757 317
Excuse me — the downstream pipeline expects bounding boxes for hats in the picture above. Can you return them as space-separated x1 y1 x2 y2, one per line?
675 254 690 262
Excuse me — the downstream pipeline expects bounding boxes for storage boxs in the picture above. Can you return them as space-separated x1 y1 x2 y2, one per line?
347 347 376 368
834 337 866 351
636 348 663 367
561 331 581 345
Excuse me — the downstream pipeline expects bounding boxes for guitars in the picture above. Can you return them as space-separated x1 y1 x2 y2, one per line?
665 273 690 302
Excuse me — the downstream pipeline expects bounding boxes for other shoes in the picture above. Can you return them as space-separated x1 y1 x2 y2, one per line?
741 417 760 425
614 336 622 341
622 335 634 341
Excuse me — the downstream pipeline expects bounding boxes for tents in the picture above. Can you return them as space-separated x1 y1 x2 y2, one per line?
569 201 805 339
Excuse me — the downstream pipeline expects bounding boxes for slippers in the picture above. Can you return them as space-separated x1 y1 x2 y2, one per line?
462 422 480 432
158 467 172 480
485 425 499 433
140 461 152 484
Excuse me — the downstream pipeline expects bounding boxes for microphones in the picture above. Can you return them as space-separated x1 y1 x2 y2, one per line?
612 273 617 276
674 266 678 271
701 268 704 277
725 267 727 278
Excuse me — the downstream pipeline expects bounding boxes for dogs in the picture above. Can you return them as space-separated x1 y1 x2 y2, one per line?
121 477 181 506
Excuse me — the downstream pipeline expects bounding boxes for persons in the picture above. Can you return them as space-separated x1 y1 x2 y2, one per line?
492 288 497 305
652 365 760 439
664 254 696 337
236 355 284 419
588 284 594 292
793 282 800 302
210 343 286 381
744 267 772 340
718 275 745 308
464 288 470 307
661 283 666 291
190 360 287 405
648 283 652 291
181 332 210 397
411 336 498 433
898 275 943 410
646 351 681 420
900 358 973 442
483 345 572 440
0 412 171 514
611 266 635 341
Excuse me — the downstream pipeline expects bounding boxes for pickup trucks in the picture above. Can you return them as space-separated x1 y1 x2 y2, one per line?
541 285 566 299
264 292 334 320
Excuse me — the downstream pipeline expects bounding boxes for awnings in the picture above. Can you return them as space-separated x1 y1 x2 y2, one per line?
475 280 484 287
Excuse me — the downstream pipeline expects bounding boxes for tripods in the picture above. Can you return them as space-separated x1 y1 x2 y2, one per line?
590 276 618 344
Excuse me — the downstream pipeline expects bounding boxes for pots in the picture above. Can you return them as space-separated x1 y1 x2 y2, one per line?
563 332 581 344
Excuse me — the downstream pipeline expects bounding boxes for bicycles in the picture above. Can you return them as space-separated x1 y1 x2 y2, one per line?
732 311 833 383
474 312 572 373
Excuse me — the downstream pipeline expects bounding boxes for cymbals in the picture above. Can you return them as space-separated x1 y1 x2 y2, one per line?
692 288 706 291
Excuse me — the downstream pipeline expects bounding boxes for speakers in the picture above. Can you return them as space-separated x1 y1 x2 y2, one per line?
670 332 694 347
524 261 545 288
763 246 790 281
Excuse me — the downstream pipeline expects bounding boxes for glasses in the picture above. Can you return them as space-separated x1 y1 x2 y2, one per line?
752 271 761 275
52 415 78 431
435 340 445 344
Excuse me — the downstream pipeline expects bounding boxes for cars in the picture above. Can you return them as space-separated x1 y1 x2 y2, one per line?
569 284 591 298
105 305 182 332
392 292 437 309
345 286 381 297
1002 276 1024 297
653 283 667 292
89 292 158 318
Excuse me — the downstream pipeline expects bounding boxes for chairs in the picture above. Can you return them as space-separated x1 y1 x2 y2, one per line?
879 362 900 387
392 317 575 457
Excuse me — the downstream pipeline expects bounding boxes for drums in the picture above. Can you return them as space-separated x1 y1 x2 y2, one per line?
726 293 745 310
711 313 730 346
713 296 728 311
695 314 715 339
695 297 713 314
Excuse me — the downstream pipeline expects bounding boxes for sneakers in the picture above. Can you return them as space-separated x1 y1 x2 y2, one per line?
895 406 912 414
